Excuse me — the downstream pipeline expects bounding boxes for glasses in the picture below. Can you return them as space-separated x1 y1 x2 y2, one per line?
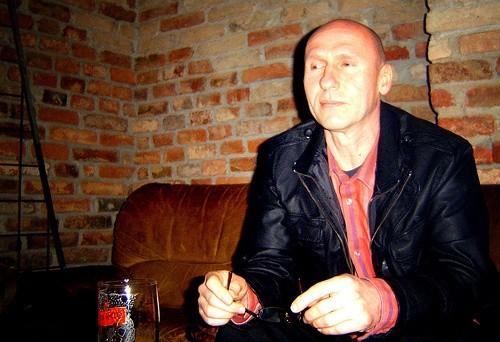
226 271 304 323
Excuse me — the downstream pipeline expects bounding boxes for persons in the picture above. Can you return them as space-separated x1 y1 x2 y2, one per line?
198 19 500 342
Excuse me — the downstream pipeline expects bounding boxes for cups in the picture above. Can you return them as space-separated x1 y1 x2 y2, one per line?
97 278 160 342
